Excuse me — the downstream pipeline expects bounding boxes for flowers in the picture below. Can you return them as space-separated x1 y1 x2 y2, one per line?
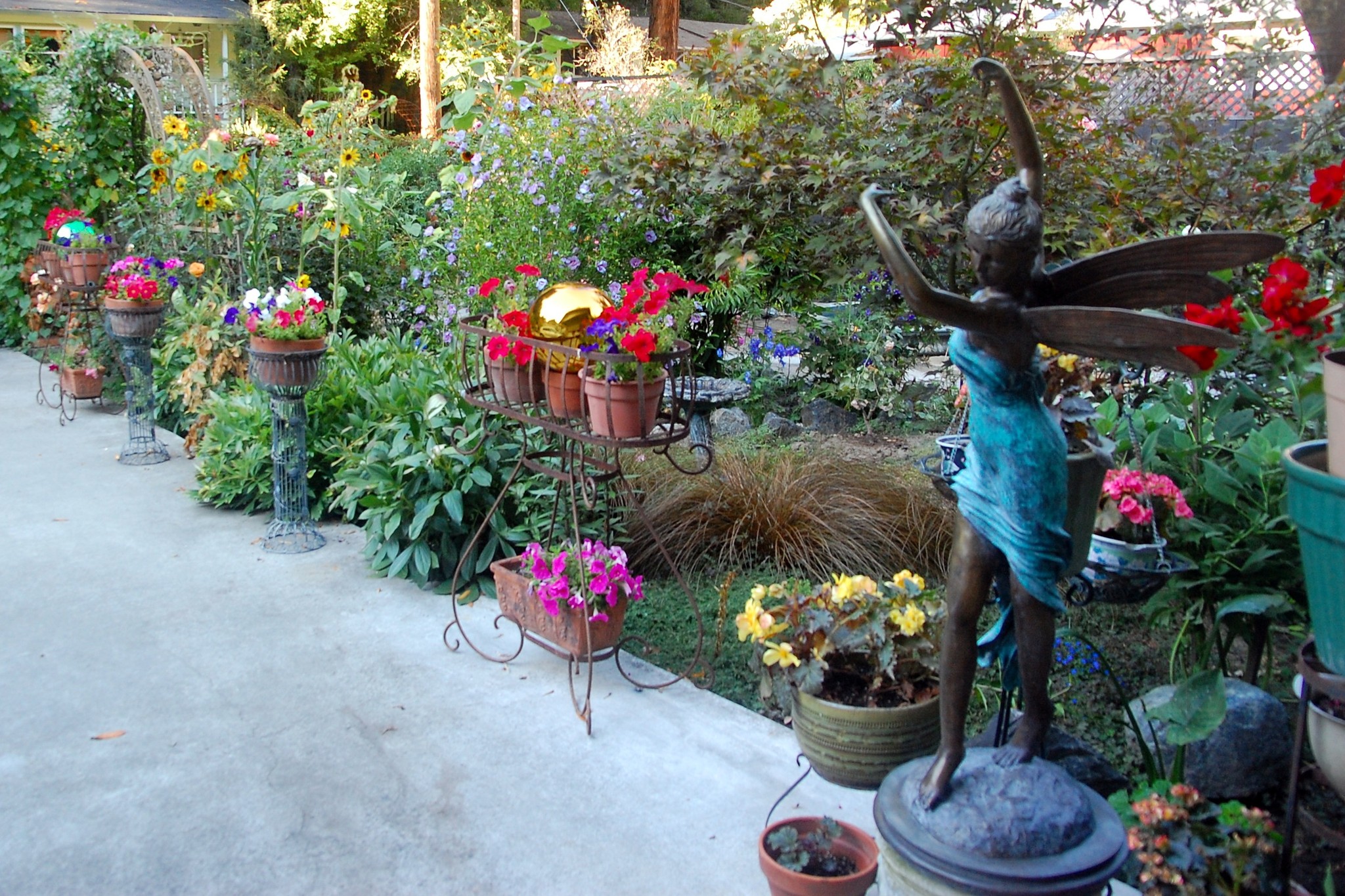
1097 468 1195 541
44 207 112 251
737 570 1282 896
480 263 709 386
105 254 184 303
1176 160 1345 370
224 274 324 339
522 538 644 623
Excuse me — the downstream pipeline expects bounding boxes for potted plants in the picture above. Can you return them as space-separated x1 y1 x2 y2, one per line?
758 817 878 896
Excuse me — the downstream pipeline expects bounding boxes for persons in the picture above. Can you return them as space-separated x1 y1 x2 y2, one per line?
858 57 1287 813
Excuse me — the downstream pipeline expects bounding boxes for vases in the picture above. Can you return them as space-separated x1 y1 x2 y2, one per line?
251 334 324 384
62 363 105 398
1283 347 1345 799
44 251 101 286
1083 536 1167 587
104 295 163 337
486 346 673 437
490 555 625 652
790 686 944 788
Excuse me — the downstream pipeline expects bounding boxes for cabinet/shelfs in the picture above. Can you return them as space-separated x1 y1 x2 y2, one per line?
31 239 130 430
444 310 703 737
